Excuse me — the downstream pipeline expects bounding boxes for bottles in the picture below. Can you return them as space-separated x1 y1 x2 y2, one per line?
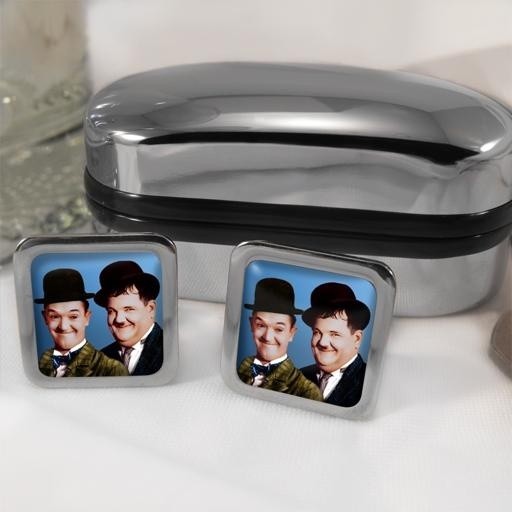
94 261 160 307
244 278 303 314
34 269 95 304
302 283 370 329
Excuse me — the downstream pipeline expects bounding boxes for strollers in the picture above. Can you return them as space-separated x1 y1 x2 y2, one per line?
250 363 278 376
52 350 75 369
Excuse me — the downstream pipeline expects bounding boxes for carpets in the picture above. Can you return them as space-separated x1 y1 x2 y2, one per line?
319 369 331 392
118 346 132 367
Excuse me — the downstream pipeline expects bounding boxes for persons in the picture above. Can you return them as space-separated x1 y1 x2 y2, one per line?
92 260 164 374
236 276 324 402
32 266 131 376
296 280 371 407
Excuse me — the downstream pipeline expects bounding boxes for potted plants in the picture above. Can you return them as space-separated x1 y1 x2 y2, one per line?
13 232 179 389
218 240 396 422
